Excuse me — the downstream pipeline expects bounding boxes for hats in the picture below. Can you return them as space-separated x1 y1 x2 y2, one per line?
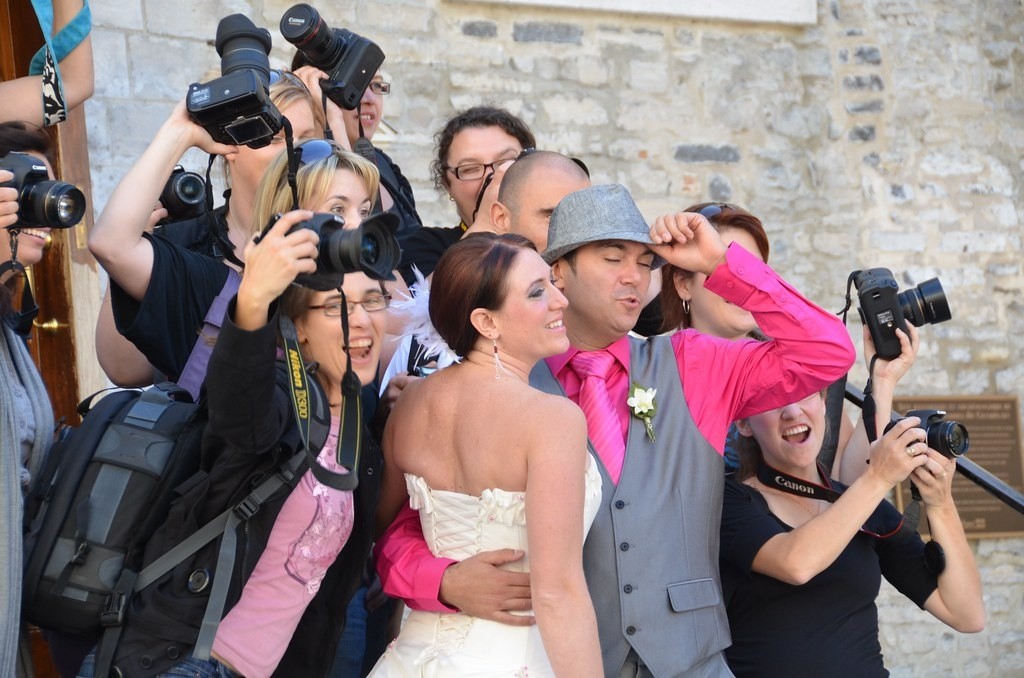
541 183 671 271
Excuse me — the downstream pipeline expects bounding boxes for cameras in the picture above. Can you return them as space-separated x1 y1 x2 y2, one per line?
883 409 970 461
855 267 952 360
264 211 401 291
0 151 86 230
279 3 386 111
157 165 208 226
186 14 284 149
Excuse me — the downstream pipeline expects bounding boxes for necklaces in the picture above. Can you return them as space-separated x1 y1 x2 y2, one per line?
783 492 822 517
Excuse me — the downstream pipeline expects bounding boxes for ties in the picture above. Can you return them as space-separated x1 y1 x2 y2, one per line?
565 351 627 488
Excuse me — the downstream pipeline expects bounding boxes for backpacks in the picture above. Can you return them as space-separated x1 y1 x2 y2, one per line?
19 365 332 640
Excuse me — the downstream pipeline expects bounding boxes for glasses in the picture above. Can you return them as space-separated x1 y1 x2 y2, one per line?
307 284 393 317
443 157 518 182
699 201 740 220
269 68 312 97
297 138 340 165
368 80 391 95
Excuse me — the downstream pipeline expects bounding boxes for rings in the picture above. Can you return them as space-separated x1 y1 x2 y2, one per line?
906 445 914 457
936 470 946 479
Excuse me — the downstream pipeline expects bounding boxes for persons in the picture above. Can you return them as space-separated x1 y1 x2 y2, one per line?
373 183 986 678
0 0 93 678
90 62 610 678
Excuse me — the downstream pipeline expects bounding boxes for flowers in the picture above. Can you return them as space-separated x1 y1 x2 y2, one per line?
626 381 658 442
